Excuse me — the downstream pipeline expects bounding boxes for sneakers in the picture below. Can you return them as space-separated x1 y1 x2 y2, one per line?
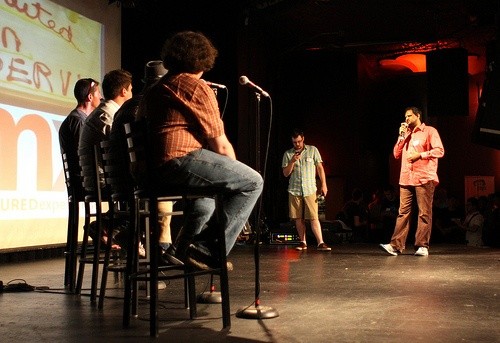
317 243 331 251
296 243 308 250
414 247 428 256
380 243 398 256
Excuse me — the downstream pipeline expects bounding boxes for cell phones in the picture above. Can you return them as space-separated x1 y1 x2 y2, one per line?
295 151 300 155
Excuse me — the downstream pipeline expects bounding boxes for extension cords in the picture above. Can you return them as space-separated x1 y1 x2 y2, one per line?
3 283 34 291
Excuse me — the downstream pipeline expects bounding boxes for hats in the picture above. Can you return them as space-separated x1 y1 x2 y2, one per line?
141 61 169 82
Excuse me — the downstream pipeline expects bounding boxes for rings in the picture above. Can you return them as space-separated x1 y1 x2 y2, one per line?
411 158 413 160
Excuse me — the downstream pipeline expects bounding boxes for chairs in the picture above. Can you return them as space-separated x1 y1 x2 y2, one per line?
62 123 231 333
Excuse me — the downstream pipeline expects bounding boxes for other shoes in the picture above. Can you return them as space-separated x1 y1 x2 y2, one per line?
84 221 102 241
187 250 233 273
165 244 184 265
100 231 122 250
135 241 148 258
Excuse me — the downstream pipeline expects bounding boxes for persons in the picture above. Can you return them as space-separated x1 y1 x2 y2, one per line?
58 32 264 271
281 132 331 252
343 184 500 246
379 107 444 256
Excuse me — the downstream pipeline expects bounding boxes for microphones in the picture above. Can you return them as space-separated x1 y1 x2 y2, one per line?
320 196 324 200
200 79 226 88
239 76 269 97
400 123 407 141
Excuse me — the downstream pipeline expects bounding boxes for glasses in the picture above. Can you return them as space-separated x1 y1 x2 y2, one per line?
88 77 96 95
